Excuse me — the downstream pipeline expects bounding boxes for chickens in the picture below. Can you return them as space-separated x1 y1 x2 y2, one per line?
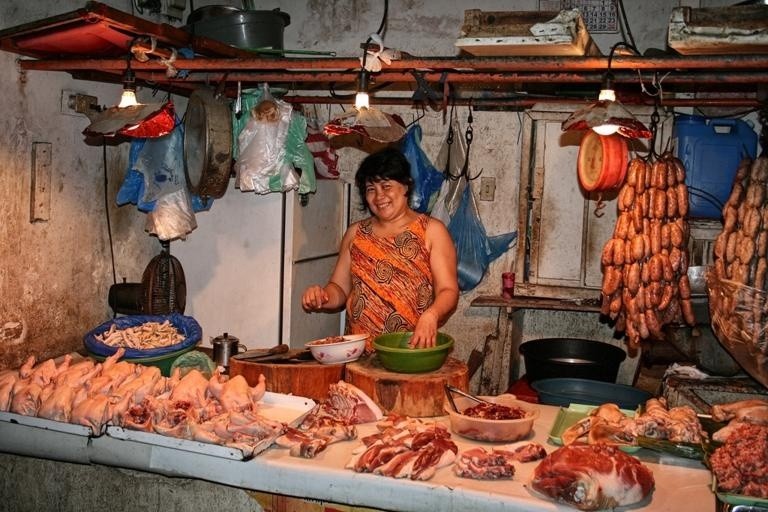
11 348 266 446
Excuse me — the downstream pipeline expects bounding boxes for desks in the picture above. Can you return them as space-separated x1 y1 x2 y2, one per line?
0 393 716 512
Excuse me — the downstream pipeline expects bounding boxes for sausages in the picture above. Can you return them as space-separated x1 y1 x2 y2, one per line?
597 154 697 352
703 154 767 384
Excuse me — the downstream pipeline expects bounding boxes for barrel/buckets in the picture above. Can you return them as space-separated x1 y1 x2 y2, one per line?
672 114 758 217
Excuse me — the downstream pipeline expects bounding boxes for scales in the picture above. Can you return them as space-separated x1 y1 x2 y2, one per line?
576 126 630 210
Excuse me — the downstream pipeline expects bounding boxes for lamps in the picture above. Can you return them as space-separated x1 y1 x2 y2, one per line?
322 1 409 146
558 42 652 143
80 35 177 141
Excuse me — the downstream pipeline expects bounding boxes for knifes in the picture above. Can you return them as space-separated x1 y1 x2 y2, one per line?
233 343 289 361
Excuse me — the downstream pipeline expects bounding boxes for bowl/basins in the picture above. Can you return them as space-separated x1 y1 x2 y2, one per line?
518 337 627 388
372 330 455 373
304 334 369 365
84 338 197 378
530 377 653 410
179 5 291 60
444 396 541 445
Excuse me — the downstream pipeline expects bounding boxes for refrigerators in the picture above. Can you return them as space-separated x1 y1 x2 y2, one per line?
162 175 350 355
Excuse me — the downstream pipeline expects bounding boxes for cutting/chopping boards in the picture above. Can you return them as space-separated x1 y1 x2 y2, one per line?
342 351 471 418
226 347 347 406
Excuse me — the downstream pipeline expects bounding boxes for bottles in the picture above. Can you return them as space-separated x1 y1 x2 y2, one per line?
502 272 514 300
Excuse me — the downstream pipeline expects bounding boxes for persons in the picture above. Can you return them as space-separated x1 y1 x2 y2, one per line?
301 146 459 352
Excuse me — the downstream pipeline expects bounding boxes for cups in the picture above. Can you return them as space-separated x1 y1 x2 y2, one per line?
213 332 247 368
